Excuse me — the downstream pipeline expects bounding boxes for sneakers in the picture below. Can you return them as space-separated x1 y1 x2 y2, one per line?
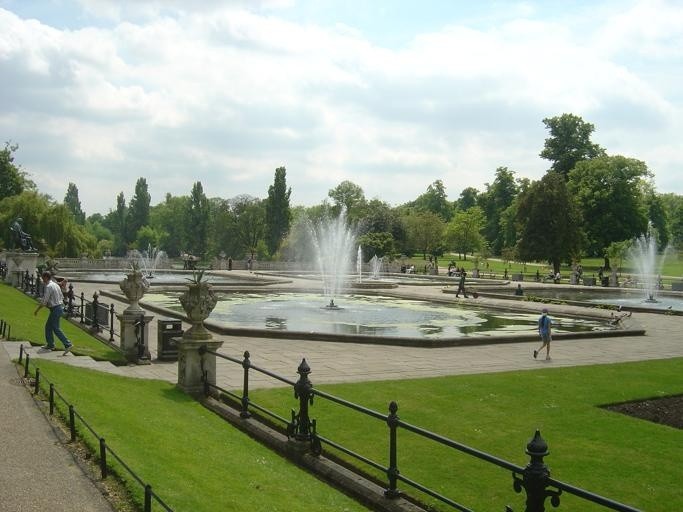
456 296 469 298
63 344 74 356
533 350 552 360
41 345 57 351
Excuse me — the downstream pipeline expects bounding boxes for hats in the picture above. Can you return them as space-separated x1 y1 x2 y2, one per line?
542 307 549 316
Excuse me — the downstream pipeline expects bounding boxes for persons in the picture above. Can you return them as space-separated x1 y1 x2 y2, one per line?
532 308 553 360
247 256 252 273
12 217 38 253
596 266 603 281
655 273 664 290
455 272 468 298
514 284 524 295
226 256 232 271
32 270 74 356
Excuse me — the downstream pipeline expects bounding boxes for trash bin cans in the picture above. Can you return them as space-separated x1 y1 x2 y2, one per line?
602 277 609 287
158 319 184 362
